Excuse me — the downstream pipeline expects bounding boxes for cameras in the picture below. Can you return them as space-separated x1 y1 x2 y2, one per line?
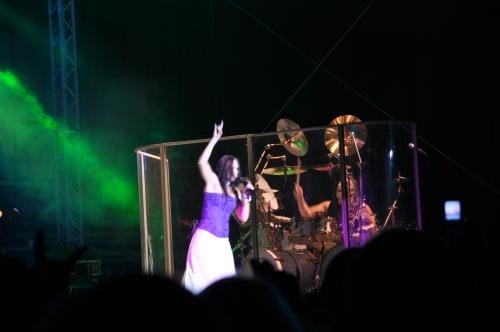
444 200 462 221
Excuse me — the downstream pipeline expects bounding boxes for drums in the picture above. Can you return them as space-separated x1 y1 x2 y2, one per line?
255 173 278 213
239 248 319 290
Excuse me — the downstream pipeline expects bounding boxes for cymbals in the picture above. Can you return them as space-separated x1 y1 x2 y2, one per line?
263 167 307 175
277 118 308 156
310 165 351 172
324 114 366 156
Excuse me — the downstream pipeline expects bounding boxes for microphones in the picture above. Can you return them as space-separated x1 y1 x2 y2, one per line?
239 177 256 195
409 143 426 155
